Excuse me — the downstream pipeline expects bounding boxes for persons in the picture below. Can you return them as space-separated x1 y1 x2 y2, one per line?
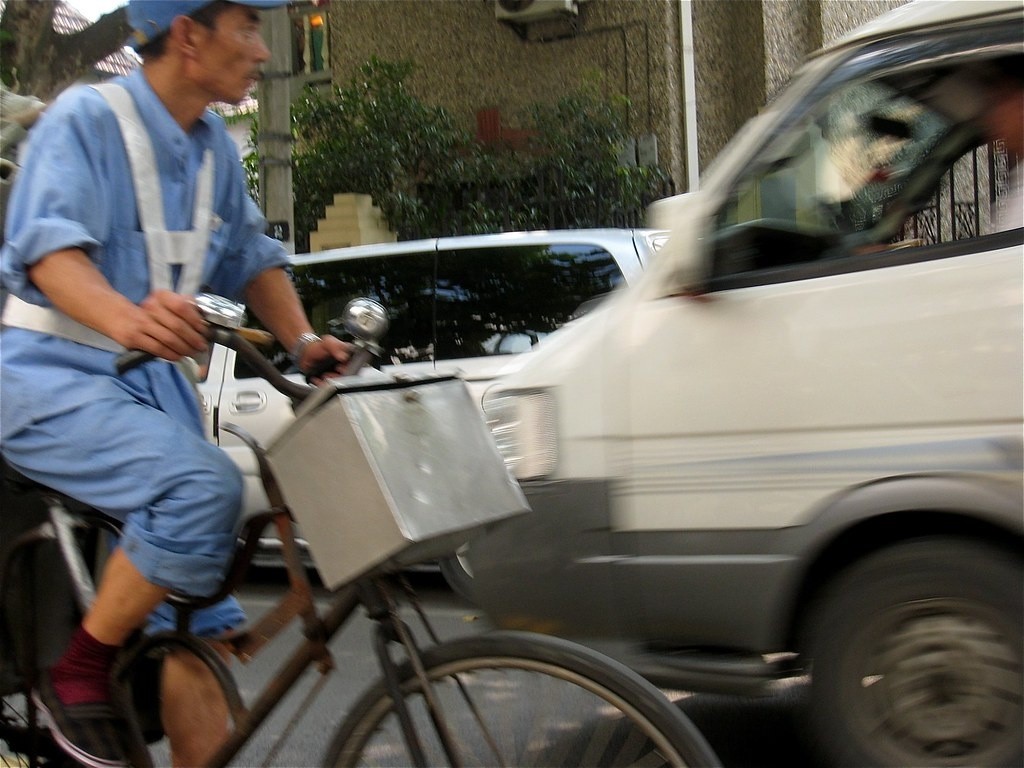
0 0 352 768
850 74 1024 261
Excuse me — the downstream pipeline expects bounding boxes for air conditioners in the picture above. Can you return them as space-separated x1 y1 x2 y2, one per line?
495 0 579 25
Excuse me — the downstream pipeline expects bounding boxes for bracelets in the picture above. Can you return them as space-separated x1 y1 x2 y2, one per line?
291 332 323 367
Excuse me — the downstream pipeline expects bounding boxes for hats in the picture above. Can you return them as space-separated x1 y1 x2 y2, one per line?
125 0 288 51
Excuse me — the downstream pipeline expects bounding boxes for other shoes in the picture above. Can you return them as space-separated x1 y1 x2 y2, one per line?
30 666 130 768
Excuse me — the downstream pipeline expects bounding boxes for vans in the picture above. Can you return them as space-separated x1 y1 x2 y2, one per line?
182 227 676 577
417 0 1024 766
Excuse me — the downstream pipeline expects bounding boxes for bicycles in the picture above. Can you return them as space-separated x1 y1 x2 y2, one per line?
1 292 727 767
